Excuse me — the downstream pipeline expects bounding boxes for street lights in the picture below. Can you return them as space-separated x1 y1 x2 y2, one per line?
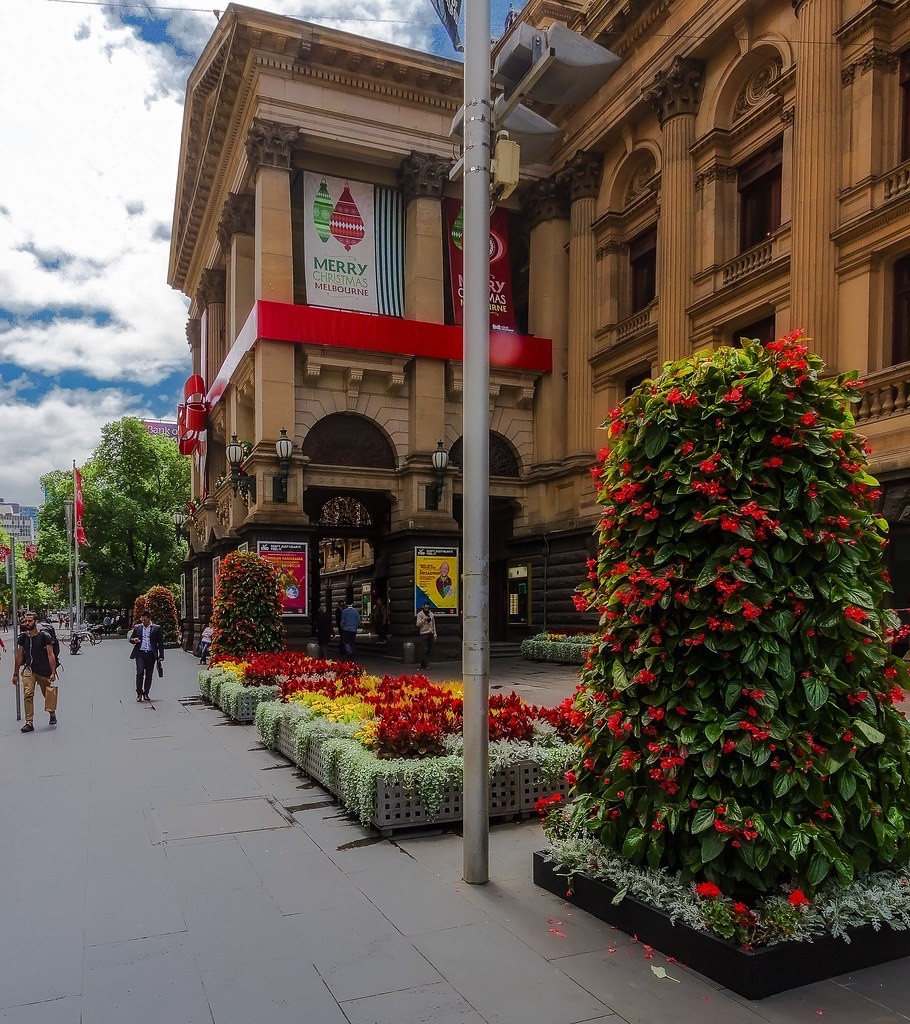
446 21 622 885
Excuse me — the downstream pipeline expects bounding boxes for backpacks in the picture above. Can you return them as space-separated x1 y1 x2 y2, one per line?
25 623 60 668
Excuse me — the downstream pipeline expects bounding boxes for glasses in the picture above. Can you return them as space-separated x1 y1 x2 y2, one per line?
339 604 343 607
424 607 430 609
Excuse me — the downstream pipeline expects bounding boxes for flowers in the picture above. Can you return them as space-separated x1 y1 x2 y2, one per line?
205 550 287 657
130 584 182 646
532 329 910 953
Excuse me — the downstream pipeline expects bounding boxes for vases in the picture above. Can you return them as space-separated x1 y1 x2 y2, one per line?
532 850 910 1003
163 645 180 649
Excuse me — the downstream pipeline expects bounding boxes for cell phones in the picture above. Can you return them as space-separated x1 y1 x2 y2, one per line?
135 638 141 642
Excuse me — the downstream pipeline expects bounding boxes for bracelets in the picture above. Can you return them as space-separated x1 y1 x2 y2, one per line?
13 673 19 677
52 673 56 678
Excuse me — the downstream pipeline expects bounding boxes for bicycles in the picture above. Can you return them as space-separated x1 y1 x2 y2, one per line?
62 625 102 647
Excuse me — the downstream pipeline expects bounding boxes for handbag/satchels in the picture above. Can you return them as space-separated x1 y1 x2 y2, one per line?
156 660 163 677
44 680 58 712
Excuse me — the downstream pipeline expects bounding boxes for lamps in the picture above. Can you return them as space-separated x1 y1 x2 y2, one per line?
225 433 256 504
172 508 190 545
272 426 293 503
425 438 449 510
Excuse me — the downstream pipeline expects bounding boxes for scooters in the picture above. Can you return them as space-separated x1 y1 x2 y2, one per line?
68 627 84 655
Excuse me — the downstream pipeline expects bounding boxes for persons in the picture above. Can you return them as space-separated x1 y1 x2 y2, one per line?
11 612 57 732
129 611 164 701
0 612 125 660
198 622 214 665
416 601 437 670
319 600 360 664
373 597 388 644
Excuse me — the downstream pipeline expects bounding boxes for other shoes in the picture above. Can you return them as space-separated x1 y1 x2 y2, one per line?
144 694 150 700
137 693 142 702
376 641 385 645
49 714 57 723
420 665 430 669
21 723 34 732
198 660 205 665
